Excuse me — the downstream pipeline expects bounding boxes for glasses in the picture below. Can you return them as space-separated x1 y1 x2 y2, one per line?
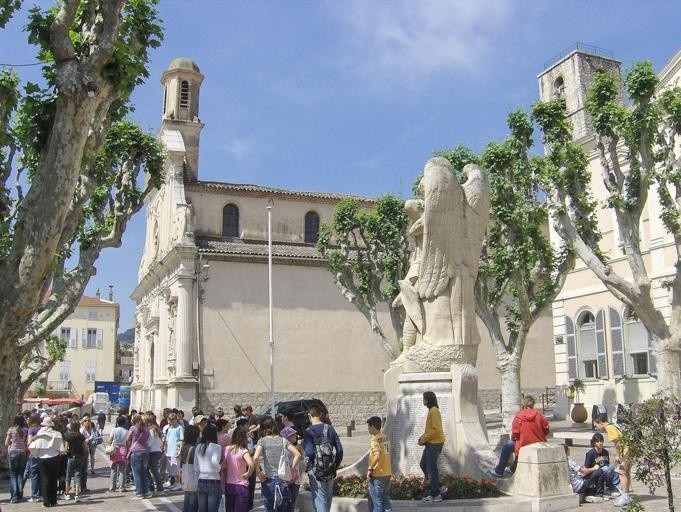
83 419 91 422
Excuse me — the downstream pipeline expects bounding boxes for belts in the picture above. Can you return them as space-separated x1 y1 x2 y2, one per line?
199 479 220 483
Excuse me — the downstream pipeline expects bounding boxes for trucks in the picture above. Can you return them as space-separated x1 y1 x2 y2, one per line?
119 385 131 407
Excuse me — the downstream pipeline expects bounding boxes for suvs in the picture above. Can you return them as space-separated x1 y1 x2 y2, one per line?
263 397 334 437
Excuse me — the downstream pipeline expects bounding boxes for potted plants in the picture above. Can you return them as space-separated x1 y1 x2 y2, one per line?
569 378 588 423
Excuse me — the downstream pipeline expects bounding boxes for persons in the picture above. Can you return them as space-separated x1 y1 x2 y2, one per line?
593 417 633 507
367 416 393 512
108 405 343 512
98 411 106 436
4 405 100 507
490 396 550 478
417 391 448 503
584 433 622 496
559 444 610 501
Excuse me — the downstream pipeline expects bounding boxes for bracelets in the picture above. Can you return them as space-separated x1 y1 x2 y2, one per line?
368 468 373 471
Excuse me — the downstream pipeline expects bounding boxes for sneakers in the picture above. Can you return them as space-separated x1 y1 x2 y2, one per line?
505 466 512 474
489 469 502 476
423 485 448 502
110 481 181 499
584 488 630 507
10 488 89 506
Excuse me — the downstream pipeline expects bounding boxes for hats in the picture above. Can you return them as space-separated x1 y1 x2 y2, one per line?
195 416 208 424
280 427 297 437
39 417 54 427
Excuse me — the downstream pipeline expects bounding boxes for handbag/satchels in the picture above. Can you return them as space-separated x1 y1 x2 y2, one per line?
182 464 199 492
105 446 116 455
278 455 292 481
92 428 103 444
273 481 292 510
120 448 131 459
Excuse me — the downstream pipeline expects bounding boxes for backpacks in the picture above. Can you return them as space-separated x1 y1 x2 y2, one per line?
305 423 336 481
